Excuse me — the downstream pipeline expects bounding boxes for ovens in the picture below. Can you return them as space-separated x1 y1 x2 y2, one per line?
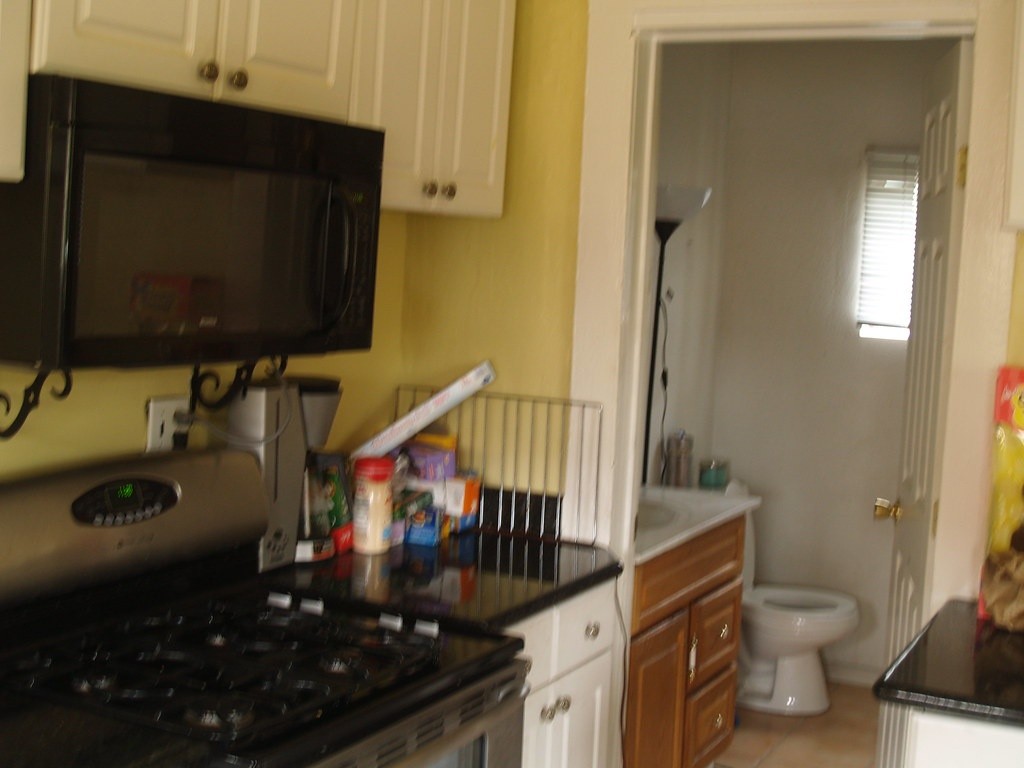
307 662 532 768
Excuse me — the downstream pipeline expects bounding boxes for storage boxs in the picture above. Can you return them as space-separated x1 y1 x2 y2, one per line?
409 434 456 480
398 475 481 536
348 359 497 470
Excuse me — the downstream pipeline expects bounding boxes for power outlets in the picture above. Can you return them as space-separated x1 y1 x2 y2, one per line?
146 394 198 456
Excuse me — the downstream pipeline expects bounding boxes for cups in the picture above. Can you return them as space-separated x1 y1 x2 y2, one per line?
665 435 695 487
699 459 729 489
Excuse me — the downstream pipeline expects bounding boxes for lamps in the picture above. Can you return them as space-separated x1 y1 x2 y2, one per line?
636 182 714 487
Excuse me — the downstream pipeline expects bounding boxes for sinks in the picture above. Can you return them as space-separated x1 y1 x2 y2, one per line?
637 493 691 534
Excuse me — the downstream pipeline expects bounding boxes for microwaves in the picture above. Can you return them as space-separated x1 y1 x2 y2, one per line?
0 73 386 367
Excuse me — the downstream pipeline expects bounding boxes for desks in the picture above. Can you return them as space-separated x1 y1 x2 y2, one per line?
879 597 1024 768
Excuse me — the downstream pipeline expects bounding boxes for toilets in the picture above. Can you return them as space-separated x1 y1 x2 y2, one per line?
723 476 860 715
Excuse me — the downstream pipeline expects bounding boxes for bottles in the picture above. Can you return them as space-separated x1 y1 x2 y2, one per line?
352 457 394 553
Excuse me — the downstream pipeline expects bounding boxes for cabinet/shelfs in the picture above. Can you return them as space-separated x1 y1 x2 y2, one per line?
320 661 532 768
626 513 751 768
509 580 619 768
0 0 517 219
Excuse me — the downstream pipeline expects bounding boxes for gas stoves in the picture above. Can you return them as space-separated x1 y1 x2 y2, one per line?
0 447 529 768
